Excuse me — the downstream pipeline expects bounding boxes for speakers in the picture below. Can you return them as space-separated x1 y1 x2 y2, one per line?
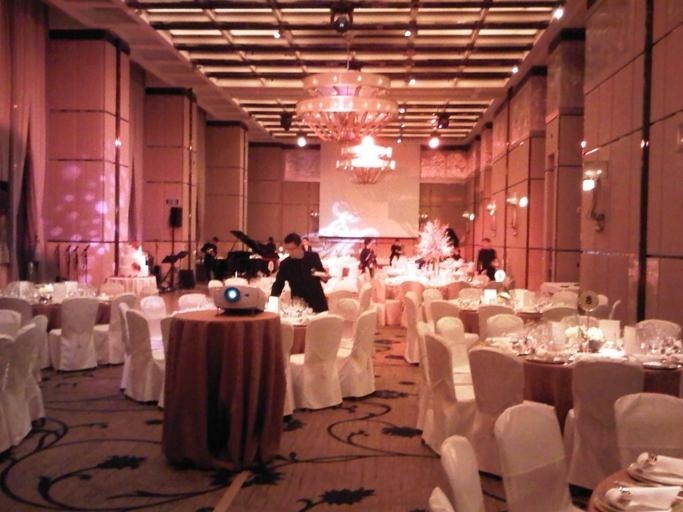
179 269 195 289
170 207 182 228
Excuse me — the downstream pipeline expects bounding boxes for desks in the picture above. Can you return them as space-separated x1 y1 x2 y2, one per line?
165 309 284 472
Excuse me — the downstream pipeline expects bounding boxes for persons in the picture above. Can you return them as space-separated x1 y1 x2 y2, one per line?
389 239 403 266
476 238 497 274
201 242 219 282
485 256 499 280
264 233 330 313
251 236 290 282
302 237 312 253
444 228 461 260
147 255 162 284
359 238 384 273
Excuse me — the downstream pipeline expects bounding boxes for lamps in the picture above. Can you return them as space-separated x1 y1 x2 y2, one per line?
506 192 518 238
296 33 399 146
405 72 415 87
429 132 440 150
486 201 498 231
399 103 406 116
512 59 519 75
296 129 309 149
581 168 608 236
461 212 475 234
334 136 396 185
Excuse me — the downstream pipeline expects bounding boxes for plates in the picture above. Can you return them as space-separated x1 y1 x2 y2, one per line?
593 485 675 512
488 334 682 370
626 455 683 488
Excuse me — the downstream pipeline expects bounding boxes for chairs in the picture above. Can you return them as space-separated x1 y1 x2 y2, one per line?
1 309 47 451
401 253 682 512
116 252 402 420
1 251 159 373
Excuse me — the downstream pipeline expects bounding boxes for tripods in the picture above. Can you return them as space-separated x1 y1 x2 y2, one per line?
163 228 178 289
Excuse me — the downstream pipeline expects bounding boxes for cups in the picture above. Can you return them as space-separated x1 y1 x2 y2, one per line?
3 286 98 302
277 295 316 318
456 294 546 309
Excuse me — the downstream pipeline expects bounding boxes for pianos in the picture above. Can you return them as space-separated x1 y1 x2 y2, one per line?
222 231 279 277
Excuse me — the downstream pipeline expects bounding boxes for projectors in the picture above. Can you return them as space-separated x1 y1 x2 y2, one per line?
213 286 267 312
